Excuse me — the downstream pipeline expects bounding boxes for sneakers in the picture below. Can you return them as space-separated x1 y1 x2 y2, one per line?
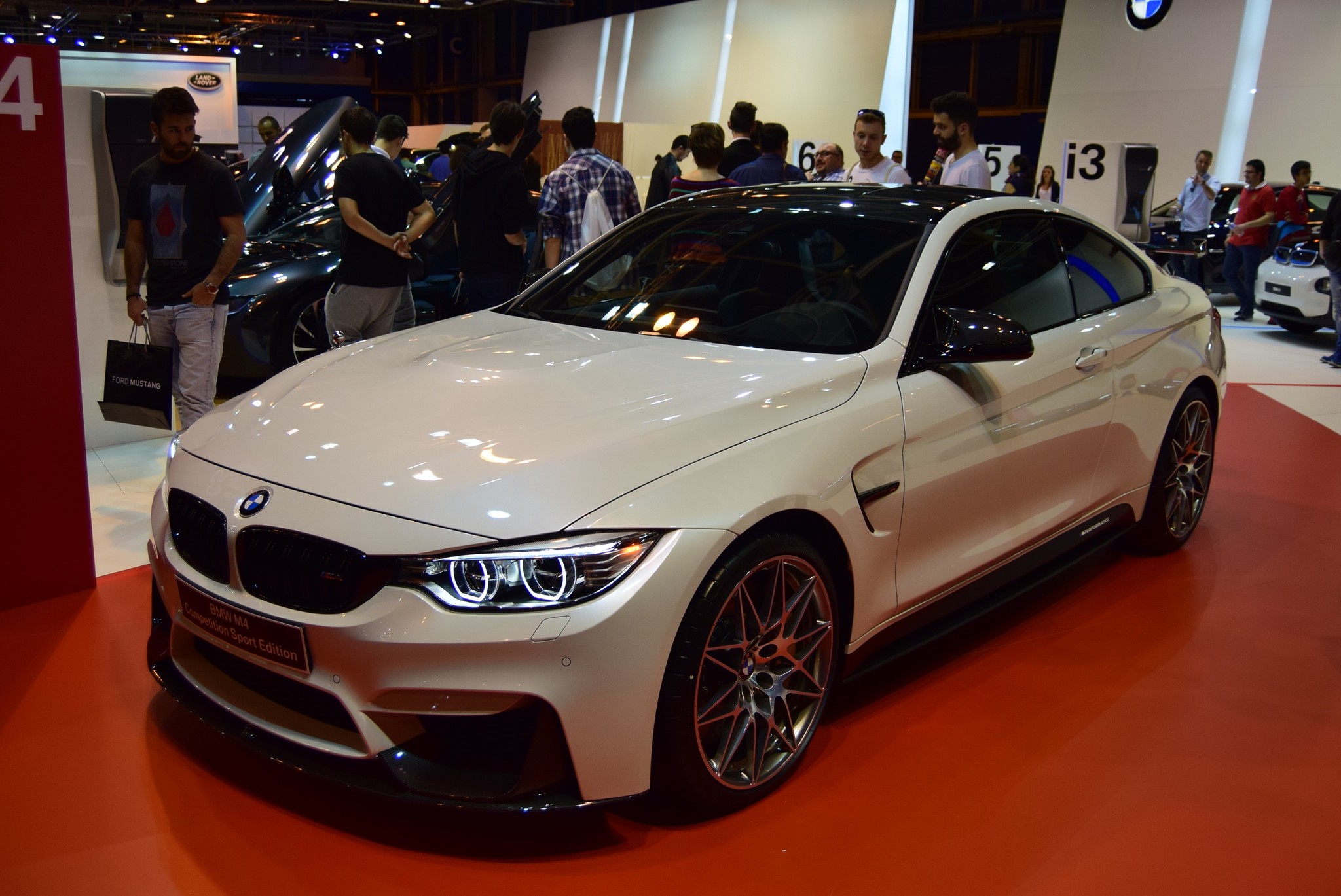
1321 354 1334 363
1329 356 1341 367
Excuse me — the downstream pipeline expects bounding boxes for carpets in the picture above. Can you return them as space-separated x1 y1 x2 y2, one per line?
0 384 1341 896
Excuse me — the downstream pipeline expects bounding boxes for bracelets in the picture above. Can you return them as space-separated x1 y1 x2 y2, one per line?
1201 178 1206 185
126 293 141 301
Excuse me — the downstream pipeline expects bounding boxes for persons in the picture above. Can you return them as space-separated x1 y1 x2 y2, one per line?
1319 190 1341 367
1170 149 1222 294
891 150 909 175
713 101 762 181
1034 165 1060 204
1220 160 1277 322
452 100 529 315
119 86 247 434
367 113 418 332
1001 153 1032 196
1271 160 1313 249
664 120 742 283
917 92 991 190
806 141 848 182
325 106 437 347
840 109 913 187
537 106 644 288
428 139 450 184
248 116 283 174
728 122 809 188
645 134 693 211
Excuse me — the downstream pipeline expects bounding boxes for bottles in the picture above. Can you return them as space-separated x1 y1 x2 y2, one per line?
1174 205 1181 218
1227 220 1244 237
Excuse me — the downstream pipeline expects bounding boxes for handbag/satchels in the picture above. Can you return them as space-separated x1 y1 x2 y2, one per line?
97 321 172 430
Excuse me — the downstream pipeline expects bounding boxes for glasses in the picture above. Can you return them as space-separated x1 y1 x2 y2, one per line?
858 109 885 118
1244 170 1256 174
815 150 840 157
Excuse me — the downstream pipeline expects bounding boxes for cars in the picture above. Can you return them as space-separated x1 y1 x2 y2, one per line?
145 180 1231 827
201 88 564 376
1137 180 1335 332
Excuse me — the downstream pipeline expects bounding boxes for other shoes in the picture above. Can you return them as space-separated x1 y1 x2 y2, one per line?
1235 307 1242 315
1236 313 1253 321
1268 317 1276 324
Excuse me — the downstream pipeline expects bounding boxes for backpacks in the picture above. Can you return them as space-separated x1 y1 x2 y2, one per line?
561 160 614 250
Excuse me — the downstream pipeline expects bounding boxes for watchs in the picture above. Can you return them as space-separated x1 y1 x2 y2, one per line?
202 279 219 294
